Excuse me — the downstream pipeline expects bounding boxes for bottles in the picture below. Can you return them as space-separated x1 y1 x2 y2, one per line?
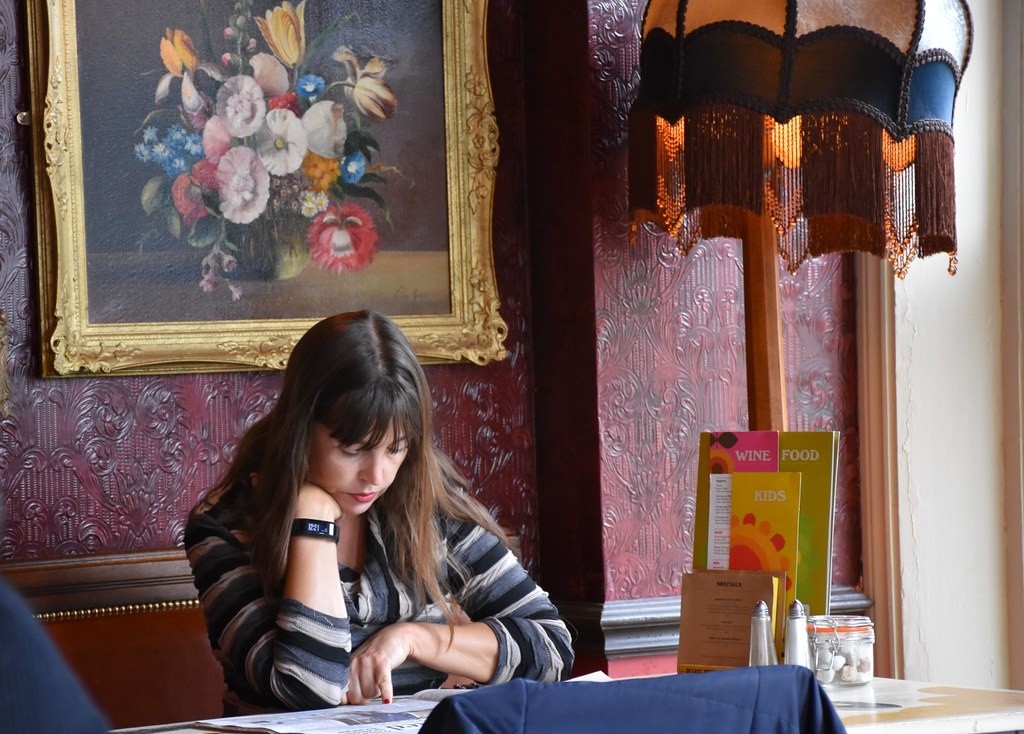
748 600 778 666
784 600 814 671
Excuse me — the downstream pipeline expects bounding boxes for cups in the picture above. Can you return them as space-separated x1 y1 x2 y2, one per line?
807 615 875 687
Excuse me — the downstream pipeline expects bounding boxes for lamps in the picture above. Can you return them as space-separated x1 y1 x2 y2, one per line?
635 0 979 666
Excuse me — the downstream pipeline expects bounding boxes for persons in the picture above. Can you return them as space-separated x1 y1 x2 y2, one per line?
183 310 575 719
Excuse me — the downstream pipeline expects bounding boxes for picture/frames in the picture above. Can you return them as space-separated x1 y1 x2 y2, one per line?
25 3 510 378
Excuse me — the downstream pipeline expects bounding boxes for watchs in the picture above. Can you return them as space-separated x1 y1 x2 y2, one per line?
290 517 339 544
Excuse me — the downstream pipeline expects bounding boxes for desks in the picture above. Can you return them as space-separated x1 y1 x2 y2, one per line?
111 643 1024 734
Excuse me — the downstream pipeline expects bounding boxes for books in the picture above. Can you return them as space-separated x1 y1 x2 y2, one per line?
675 431 841 676
